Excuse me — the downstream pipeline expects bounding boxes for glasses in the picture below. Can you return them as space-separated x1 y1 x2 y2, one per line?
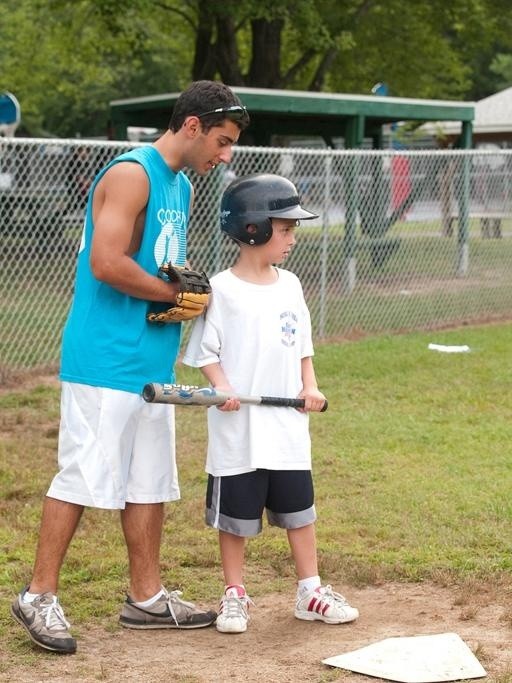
183 105 249 125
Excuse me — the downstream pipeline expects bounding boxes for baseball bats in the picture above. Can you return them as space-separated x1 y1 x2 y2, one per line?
143 383 328 412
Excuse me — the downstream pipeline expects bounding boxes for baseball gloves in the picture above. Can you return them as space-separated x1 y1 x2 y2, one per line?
146 261 212 322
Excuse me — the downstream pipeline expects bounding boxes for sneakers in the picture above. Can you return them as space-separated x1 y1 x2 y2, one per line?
118 593 217 629
294 587 359 624
216 586 249 632
11 585 77 653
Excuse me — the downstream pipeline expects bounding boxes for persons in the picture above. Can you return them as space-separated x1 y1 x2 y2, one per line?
182 170 359 635
9 80 251 655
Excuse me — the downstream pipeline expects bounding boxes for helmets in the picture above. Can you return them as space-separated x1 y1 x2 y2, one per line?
220 174 319 245
